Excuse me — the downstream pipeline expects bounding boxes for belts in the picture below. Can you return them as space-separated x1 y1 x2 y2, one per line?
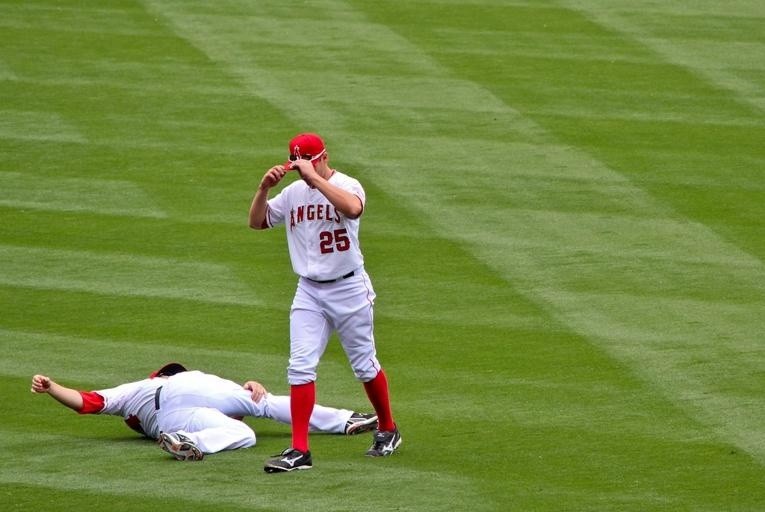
155 387 160 409
307 272 354 284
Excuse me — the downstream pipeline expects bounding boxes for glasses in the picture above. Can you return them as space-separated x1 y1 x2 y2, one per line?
289 154 316 160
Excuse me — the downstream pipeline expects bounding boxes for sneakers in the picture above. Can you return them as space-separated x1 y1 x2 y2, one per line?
345 413 378 435
264 448 312 472
158 432 203 462
366 422 402 456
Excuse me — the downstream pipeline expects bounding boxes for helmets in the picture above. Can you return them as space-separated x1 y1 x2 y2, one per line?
284 134 326 171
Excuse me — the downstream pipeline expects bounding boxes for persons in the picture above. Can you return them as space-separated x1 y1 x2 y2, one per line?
247 132 403 471
28 361 379 462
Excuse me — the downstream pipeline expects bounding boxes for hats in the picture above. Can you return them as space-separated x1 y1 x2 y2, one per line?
150 363 186 377
